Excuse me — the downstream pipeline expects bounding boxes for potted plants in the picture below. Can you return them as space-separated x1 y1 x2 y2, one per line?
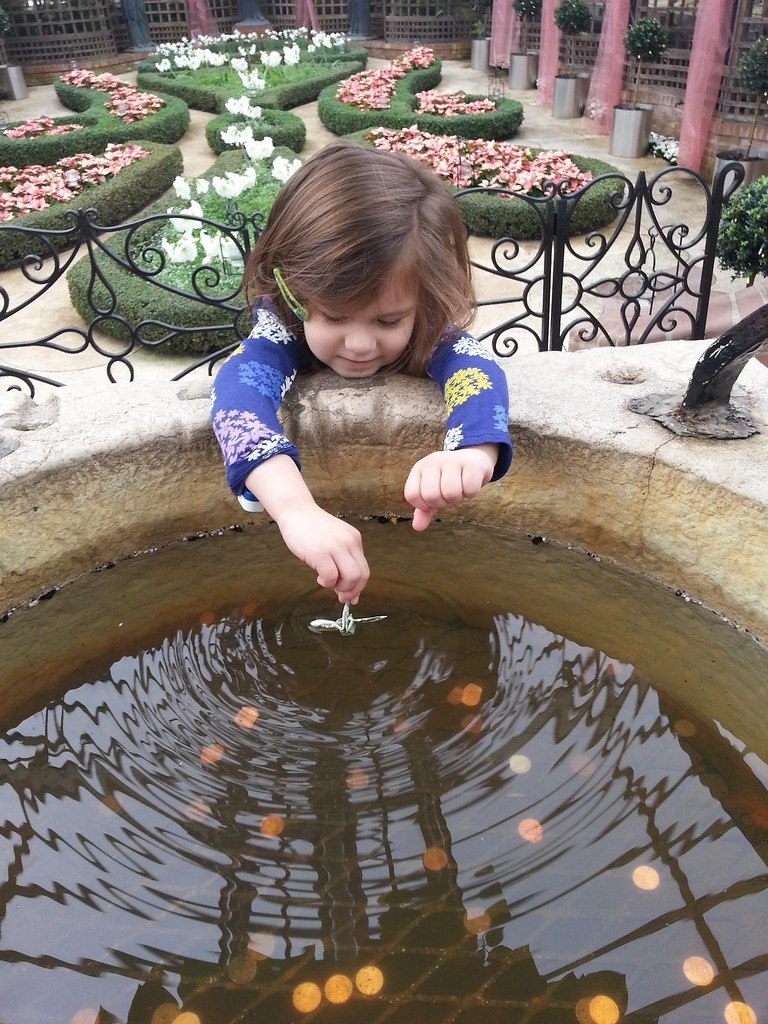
508 0 543 90
713 35 768 199
609 16 669 158
0 6 29 101
551 0 593 119
470 0 494 71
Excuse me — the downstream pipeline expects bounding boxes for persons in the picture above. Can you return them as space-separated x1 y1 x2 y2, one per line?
211 141 514 604
